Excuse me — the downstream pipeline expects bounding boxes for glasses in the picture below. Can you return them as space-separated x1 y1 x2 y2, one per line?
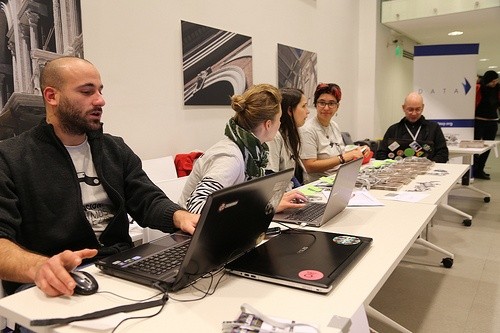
315 102 337 109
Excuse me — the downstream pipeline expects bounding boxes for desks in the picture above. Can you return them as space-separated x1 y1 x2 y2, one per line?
0 140 499 333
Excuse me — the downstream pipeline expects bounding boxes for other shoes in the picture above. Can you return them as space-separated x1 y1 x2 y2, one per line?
471 172 490 179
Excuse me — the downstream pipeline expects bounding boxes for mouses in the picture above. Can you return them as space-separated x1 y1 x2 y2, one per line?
67 271 99 295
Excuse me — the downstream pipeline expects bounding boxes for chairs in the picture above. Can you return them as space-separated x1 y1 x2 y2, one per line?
142 176 188 243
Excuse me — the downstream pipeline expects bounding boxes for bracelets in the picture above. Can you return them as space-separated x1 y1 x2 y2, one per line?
337 154 346 165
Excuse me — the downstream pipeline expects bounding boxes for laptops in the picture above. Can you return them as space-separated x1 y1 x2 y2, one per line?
92 168 293 292
270 157 366 227
222 227 375 293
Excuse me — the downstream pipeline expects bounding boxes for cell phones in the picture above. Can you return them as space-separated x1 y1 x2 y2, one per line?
266 225 281 235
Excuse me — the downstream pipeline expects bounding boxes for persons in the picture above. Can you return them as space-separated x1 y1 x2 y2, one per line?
265 87 311 191
374 92 450 164
298 83 370 174
178 84 309 213
470 70 500 180
0 56 202 298
